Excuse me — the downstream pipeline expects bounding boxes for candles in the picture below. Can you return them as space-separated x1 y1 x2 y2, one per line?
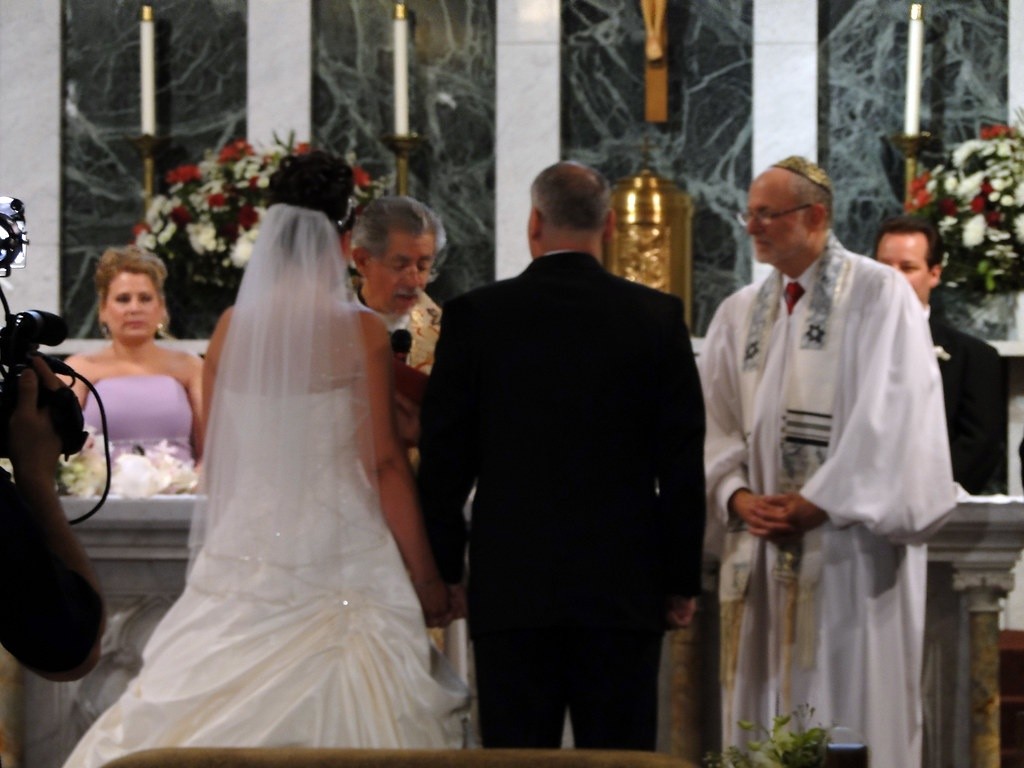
902 2 925 139
139 5 160 135
388 0 413 136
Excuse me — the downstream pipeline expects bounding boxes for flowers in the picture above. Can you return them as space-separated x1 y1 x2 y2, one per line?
137 136 388 288
909 110 1024 293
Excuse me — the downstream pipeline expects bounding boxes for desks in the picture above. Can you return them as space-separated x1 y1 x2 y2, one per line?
1 487 1023 767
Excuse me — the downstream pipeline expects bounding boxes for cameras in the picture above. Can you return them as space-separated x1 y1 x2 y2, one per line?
0 369 85 458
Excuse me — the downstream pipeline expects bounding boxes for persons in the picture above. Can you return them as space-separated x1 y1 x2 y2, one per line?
59 150 468 768
53 243 205 473
696 153 956 768
875 214 1004 493
416 161 705 754
0 358 107 682
344 195 443 473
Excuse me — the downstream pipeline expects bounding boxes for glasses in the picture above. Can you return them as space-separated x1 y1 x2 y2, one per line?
736 202 815 226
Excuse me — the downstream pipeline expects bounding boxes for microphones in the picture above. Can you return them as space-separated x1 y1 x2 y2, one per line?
15 308 69 345
391 329 413 365
